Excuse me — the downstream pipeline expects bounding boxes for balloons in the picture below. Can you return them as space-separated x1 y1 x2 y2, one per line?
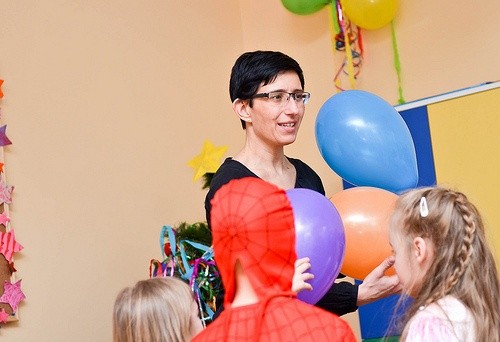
285 89 419 306
281 0 400 30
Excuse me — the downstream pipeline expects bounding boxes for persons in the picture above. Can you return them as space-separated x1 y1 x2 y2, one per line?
113 277 204 342
190 176 357 342
387 186 500 342
203 50 406 322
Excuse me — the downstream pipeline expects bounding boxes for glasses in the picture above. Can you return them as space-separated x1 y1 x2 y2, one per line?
240 91 311 104
